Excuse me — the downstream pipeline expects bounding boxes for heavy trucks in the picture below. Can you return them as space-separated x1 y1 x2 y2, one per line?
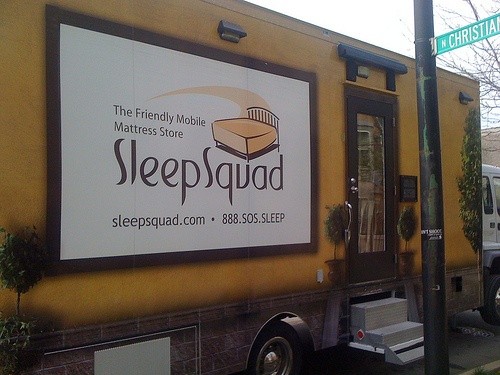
0 2 499 375
